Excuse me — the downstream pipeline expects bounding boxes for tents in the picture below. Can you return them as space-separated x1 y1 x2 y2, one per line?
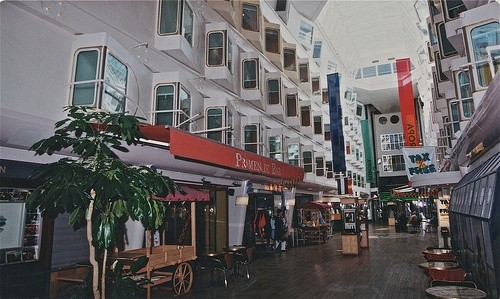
377 184 435 214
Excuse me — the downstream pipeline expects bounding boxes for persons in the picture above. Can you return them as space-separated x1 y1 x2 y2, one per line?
376 206 437 233
272 208 288 251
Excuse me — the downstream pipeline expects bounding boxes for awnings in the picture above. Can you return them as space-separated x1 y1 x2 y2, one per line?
88 123 304 201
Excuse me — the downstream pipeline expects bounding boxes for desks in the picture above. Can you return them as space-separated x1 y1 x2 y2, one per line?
425 286 488 299
423 249 451 255
304 224 328 244
419 261 459 271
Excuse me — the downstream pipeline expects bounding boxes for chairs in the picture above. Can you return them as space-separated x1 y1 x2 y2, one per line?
196 244 254 287
426 247 477 289
